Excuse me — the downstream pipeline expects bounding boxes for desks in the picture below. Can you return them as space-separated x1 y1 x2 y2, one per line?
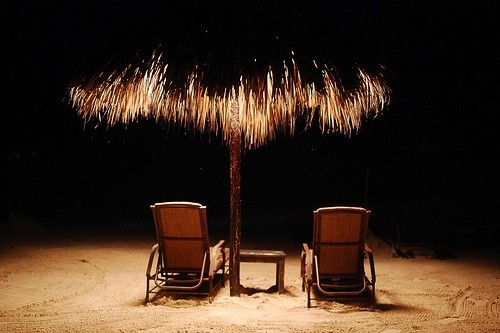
239 248 285 291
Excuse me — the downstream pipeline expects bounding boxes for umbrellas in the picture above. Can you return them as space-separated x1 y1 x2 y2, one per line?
64 20 390 296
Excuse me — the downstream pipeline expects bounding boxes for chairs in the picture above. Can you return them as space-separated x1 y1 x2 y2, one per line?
297 204 376 304
144 201 230 304
